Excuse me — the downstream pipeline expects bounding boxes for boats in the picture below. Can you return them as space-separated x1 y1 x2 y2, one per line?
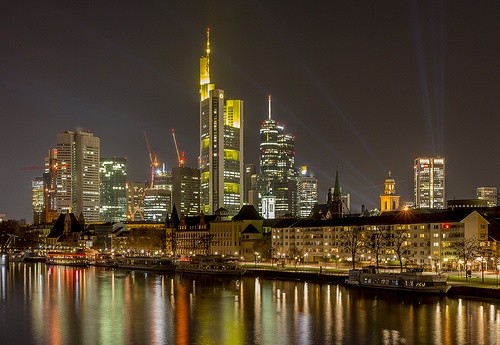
175 253 248 276
344 265 453 295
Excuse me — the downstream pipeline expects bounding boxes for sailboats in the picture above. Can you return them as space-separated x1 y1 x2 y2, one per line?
45 251 90 267
92 253 116 266
114 254 176 271
